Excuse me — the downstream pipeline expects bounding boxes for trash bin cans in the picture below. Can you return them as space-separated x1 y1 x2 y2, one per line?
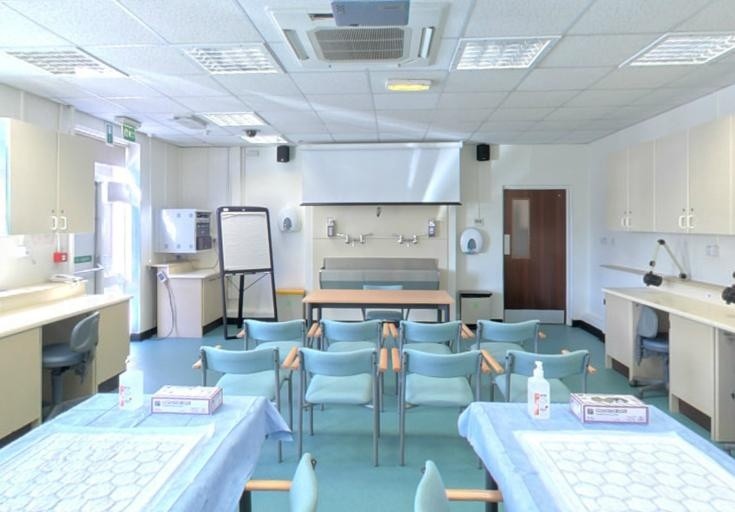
457 290 492 329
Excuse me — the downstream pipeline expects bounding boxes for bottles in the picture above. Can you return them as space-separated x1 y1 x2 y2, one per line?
116 355 147 414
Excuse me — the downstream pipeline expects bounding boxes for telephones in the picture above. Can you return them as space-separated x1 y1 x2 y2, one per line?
49 273 83 282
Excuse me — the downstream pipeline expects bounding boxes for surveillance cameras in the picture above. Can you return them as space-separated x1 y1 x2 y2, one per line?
247 130 256 137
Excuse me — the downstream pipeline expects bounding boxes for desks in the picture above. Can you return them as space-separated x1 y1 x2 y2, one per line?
457 398 735 512
0 387 293 512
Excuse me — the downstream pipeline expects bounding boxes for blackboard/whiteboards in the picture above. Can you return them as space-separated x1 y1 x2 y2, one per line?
217 206 273 275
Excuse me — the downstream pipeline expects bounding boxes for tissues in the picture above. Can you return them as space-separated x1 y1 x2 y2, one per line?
151 385 223 414
569 394 650 425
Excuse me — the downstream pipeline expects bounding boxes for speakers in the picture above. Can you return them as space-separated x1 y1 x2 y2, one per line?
277 146 289 162
477 144 490 161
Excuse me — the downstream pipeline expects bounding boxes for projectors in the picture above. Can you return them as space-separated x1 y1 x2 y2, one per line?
331 0 409 26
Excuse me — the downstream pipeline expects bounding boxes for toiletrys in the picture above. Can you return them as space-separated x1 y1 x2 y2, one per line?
118 356 144 410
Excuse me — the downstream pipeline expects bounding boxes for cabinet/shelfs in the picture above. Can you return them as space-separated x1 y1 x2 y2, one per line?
602 289 666 387
655 114 735 237
152 259 228 337
0 284 135 443
1 118 96 234
667 313 735 442
605 137 655 232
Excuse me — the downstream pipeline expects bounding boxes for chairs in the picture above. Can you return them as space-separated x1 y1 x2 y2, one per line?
414 458 505 512
635 307 668 396
245 453 319 512
43 311 100 415
